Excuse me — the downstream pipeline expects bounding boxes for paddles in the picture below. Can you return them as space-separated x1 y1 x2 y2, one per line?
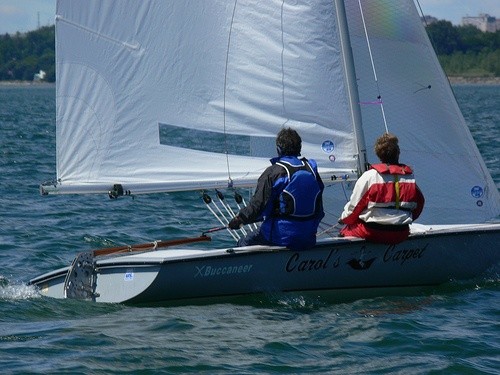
89 225 230 257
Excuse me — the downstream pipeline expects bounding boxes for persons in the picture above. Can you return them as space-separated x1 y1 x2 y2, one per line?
229 127 325 251
337 133 425 245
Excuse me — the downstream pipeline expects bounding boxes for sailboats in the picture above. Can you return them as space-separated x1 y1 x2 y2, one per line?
25 0 500 303
